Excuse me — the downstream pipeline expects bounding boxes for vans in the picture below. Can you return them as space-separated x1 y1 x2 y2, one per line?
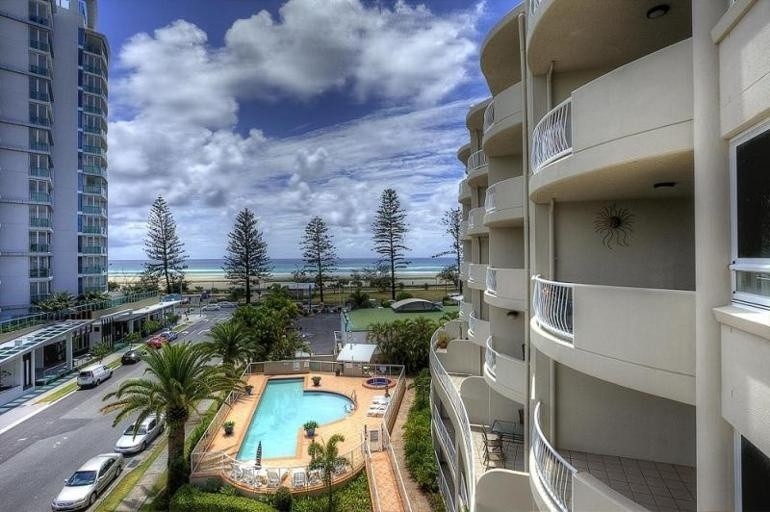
76 364 114 389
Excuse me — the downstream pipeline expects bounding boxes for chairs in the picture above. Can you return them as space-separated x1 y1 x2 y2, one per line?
231 458 319 490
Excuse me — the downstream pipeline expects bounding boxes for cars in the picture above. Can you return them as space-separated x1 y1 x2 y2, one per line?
49 451 125 511
215 301 239 309
120 349 143 365
158 292 188 309
159 331 178 342
113 413 166 456
201 304 221 311
145 337 164 349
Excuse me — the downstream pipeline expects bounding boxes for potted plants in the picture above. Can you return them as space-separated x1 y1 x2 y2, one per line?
302 419 320 437
221 419 235 434
